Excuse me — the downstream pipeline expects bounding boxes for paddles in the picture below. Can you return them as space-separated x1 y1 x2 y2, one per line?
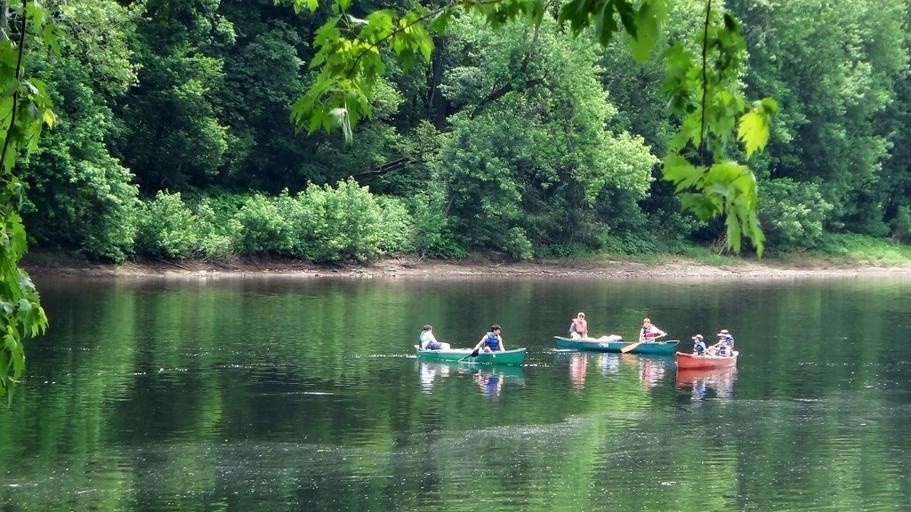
621 332 667 353
460 349 478 361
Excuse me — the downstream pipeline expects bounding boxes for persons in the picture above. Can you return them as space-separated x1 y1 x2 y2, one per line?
716 368 736 404
473 324 506 353
638 318 668 343
688 375 709 404
713 329 736 358
639 357 667 388
569 311 590 341
476 370 506 401
420 363 437 396
692 333 708 355
419 324 446 351
567 351 591 391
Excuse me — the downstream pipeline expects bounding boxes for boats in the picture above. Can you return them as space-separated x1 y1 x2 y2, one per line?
553 334 680 355
413 344 527 367
674 350 741 371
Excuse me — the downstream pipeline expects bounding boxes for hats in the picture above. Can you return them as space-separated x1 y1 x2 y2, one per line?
717 330 731 337
691 334 703 339
